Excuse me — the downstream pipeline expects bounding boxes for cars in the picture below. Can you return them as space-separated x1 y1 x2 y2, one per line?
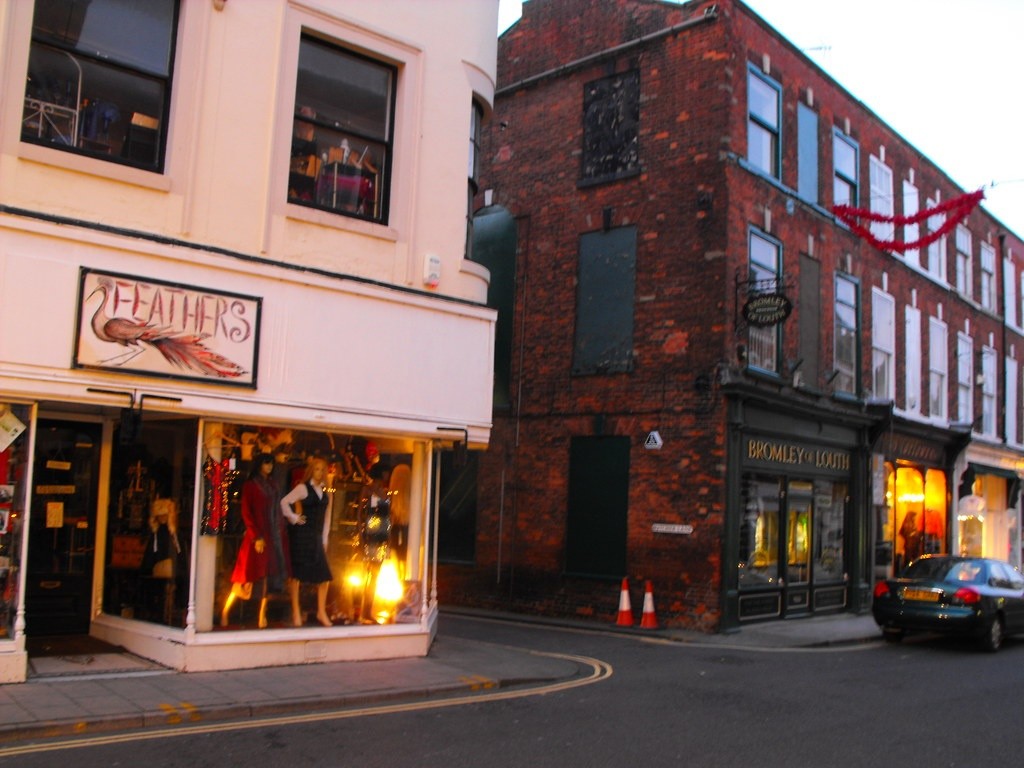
870 554 1024 651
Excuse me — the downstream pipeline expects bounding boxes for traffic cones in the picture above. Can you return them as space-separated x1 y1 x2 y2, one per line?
638 581 662 630
613 576 637 627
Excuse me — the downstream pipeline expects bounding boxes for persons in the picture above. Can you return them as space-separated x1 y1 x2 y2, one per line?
145 482 181 577
898 512 920 562
280 458 333 628
220 453 282 629
